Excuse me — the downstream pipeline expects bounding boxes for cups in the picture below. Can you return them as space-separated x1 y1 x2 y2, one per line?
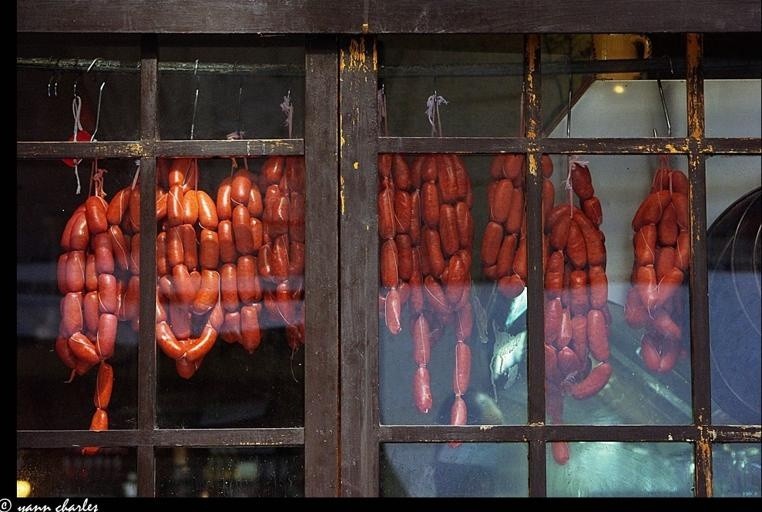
589 33 651 80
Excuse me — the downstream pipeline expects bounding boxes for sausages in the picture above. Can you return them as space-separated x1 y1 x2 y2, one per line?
624 171 691 372
55 196 131 375
155 158 224 378
217 168 265 356
482 154 554 297
83 408 109 455
260 156 304 349
548 417 571 466
411 154 481 340
93 362 115 408
379 153 433 415
450 396 467 449
107 182 166 379
453 341 471 396
544 162 612 416
